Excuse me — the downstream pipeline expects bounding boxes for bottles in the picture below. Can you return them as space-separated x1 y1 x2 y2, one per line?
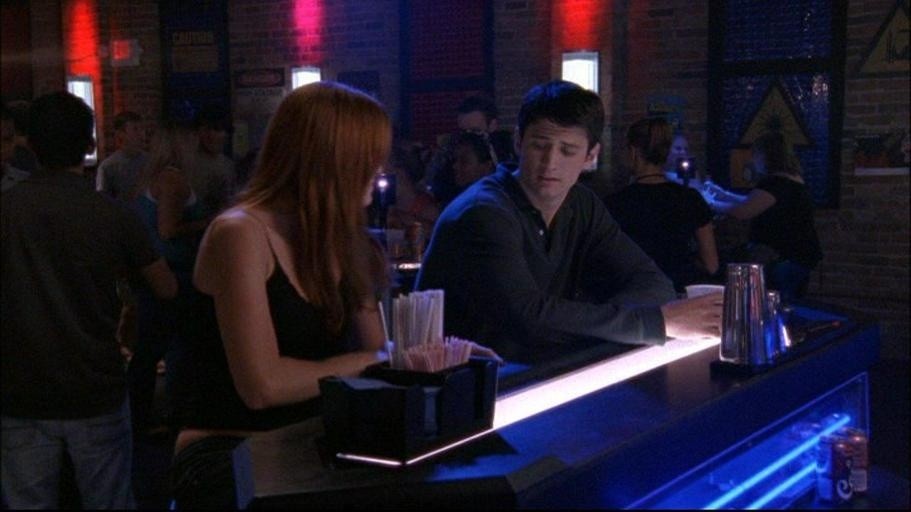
719 263 766 365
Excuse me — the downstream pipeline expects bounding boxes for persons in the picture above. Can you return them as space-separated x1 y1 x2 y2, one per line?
415 77 724 344
3 90 178 511
711 127 818 313
155 81 505 510
1 92 510 404
602 116 724 299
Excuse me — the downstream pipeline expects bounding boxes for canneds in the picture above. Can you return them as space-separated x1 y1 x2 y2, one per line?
406 221 425 256
